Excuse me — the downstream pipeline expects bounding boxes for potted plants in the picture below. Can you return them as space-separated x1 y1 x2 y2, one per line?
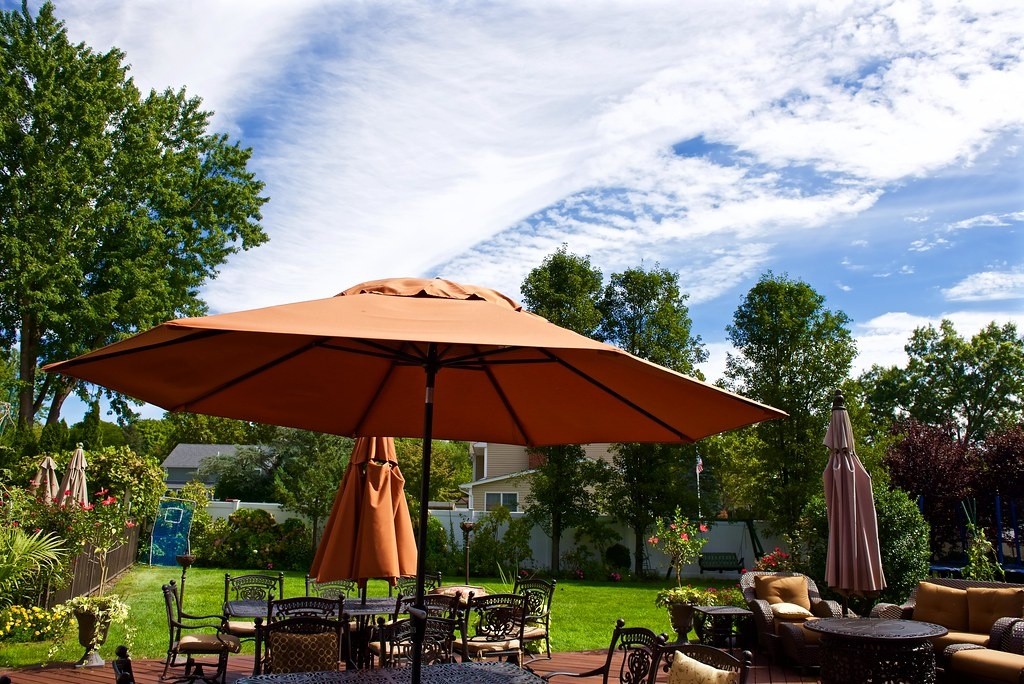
41 598 136 669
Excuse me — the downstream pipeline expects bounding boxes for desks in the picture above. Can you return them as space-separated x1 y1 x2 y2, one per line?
225 597 442 668
234 662 546 684
803 618 949 684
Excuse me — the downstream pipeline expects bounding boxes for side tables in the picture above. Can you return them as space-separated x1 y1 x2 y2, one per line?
692 606 753 654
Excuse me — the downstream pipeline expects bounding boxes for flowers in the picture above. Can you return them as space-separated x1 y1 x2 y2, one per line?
656 585 719 607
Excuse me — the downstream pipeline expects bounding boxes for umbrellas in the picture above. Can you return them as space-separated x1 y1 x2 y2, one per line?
39 275 792 683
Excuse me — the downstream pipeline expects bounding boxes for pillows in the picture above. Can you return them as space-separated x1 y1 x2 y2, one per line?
771 602 815 619
754 575 810 611
965 587 1024 635
669 650 738 684
912 581 968 633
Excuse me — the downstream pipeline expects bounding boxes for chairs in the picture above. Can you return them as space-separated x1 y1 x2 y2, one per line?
252 612 352 675
267 594 346 624
389 571 442 597
305 574 361 599
220 572 284 674
377 610 473 668
369 590 463 670
648 635 754 684
476 575 556 659
452 590 532 666
543 618 669 684
161 580 242 684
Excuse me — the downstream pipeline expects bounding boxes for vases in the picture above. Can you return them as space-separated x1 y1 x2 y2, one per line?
669 604 697 646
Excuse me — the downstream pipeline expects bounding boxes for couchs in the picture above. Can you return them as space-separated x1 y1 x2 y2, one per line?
942 622 1024 683
740 571 842 670
870 578 1024 674
